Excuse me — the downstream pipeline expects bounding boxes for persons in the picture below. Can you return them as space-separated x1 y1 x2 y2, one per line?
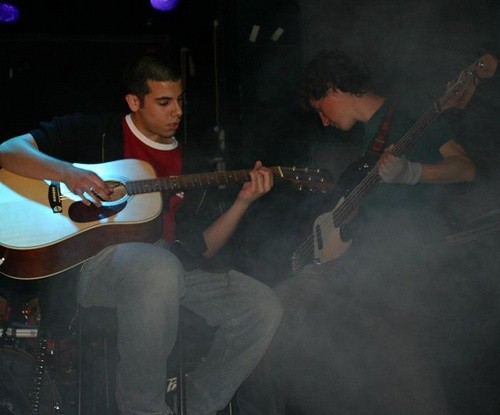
234 49 479 414
0 50 283 415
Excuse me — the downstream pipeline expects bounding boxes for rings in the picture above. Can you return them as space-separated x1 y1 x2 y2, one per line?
90 184 95 190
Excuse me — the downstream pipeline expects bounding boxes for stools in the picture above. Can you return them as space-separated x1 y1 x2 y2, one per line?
61 304 188 415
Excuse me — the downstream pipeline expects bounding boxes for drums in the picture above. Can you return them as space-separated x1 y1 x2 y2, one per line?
37 336 72 367
0 346 64 415
20 296 41 326
0 293 12 325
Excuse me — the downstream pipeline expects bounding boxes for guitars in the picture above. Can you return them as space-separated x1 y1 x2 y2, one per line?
0 146 336 280
282 53 498 275
